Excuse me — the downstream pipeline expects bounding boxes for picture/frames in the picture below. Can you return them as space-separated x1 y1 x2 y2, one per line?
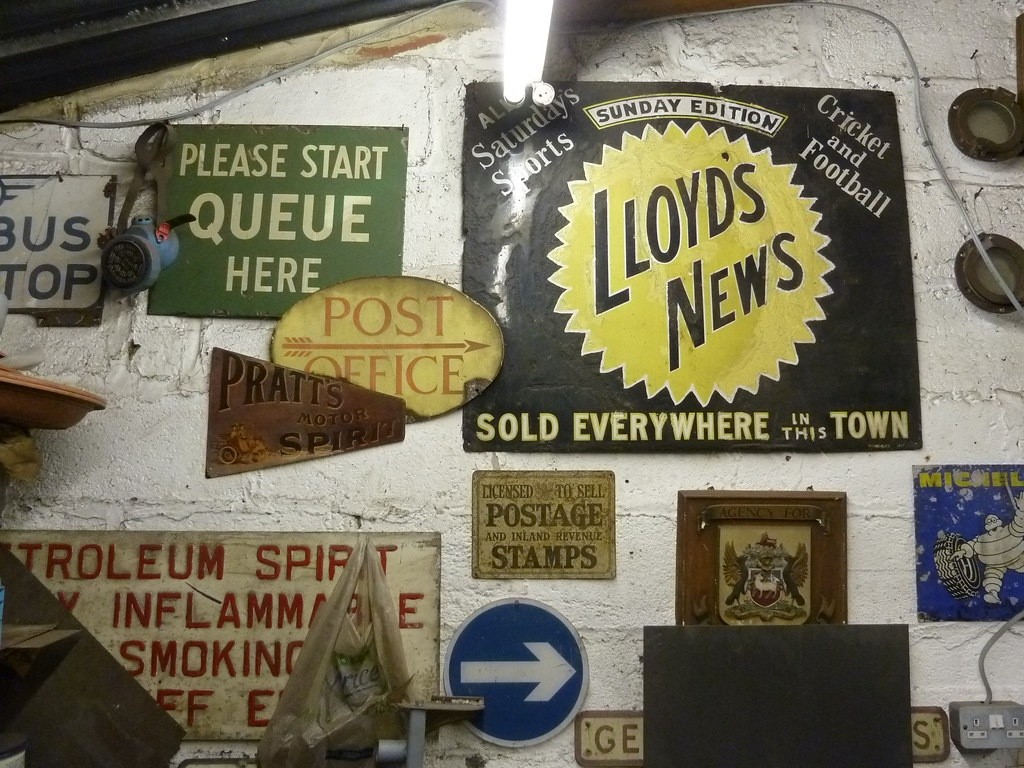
676 488 849 625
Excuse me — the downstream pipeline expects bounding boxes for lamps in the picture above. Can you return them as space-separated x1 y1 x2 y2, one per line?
500 0 553 111
